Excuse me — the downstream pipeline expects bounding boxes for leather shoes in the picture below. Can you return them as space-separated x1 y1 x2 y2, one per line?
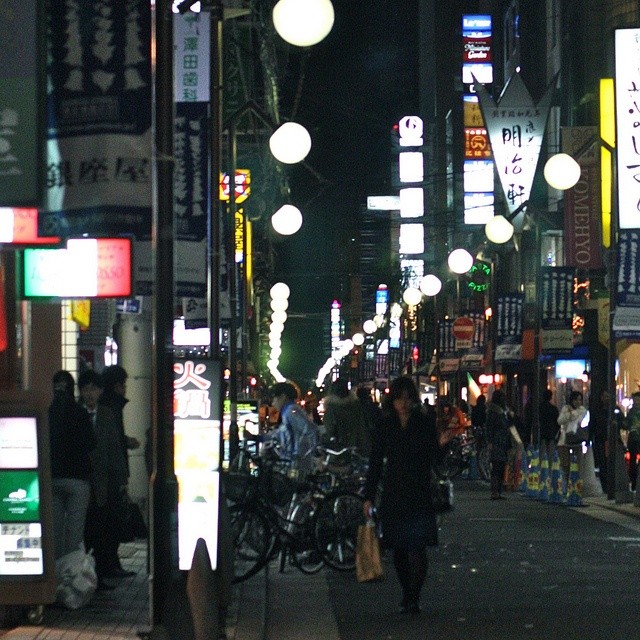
107 566 136 577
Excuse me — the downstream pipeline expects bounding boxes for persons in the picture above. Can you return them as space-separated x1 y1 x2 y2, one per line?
486 390 516 501
237 378 317 497
303 397 322 425
98 365 140 577
438 395 464 451
556 389 591 486
589 387 626 493
471 394 486 428
323 377 373 465
77 369 129 589
362 375 446 616
619 392 640 494
354 382 380 423
47 367 97 592
538 390 559 472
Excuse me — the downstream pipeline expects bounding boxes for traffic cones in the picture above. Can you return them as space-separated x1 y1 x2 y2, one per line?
517 447 530 497
524 445 541 497
539 439 553 504
549 448 568 504
563 454 588 507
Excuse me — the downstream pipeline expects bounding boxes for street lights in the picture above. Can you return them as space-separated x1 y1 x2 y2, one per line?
419 278 465 411
211 1 337 623
241 186 305 393
229 102 311 468
448 248 496 446
543 136 616 500
486 200 540 448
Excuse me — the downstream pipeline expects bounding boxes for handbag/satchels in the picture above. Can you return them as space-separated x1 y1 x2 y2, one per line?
118 488 134 543
507 420 522 448
131 501 147 539
355 512 383 583
429 456 455 514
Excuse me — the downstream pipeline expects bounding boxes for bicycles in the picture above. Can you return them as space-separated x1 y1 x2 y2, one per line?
440 426 492 481
232 420 383 581
